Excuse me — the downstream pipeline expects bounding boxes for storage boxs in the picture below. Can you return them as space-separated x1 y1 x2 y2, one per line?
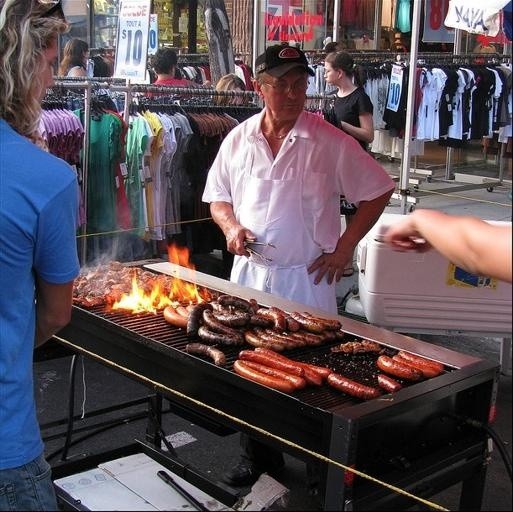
354 218 509 329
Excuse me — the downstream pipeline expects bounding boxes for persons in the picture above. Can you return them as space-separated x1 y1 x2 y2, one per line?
0 1 82 508
57 39 90 80
318 50 376 239
200 45 396 319
213 74 246 105
142 47 197 97
384 201 513 288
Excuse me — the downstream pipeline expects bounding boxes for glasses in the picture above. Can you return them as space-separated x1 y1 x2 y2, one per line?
37 0 67 24
266 79 308 93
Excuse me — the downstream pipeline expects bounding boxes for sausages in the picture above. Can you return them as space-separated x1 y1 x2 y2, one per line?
164 295 443 399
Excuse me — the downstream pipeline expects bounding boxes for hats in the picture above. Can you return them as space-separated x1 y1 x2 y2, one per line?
254 44 315 78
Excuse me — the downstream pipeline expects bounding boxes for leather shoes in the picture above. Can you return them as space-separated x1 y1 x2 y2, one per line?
223 462 260 486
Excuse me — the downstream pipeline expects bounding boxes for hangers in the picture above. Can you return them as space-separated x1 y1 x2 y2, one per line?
300 50 512 73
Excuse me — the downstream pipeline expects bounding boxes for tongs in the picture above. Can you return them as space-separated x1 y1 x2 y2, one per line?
242 241 275 263
